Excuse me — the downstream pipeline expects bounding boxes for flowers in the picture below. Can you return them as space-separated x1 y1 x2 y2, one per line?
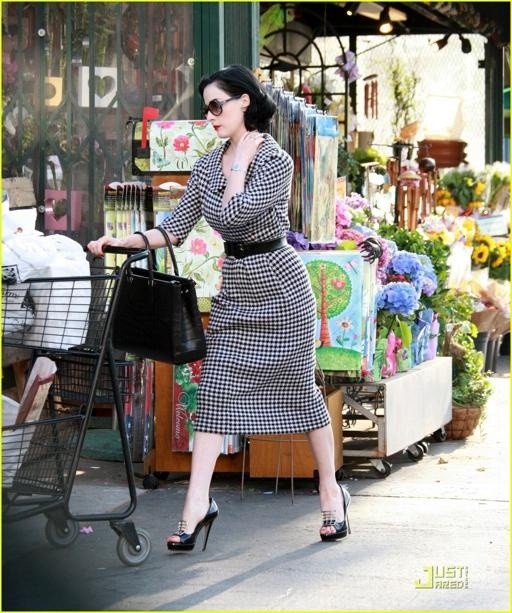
326 145 509 338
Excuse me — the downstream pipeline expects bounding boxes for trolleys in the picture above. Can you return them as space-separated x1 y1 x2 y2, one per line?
2 247 150 566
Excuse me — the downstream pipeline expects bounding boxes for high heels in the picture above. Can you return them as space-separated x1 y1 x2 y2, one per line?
320 483 353 541
167 496 220 553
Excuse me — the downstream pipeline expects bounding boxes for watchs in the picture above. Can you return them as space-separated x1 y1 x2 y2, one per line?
230 162 248 173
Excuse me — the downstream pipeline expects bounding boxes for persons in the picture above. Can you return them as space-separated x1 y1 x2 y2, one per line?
86 64 353 551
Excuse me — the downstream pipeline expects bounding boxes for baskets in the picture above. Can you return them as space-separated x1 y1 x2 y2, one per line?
445 404 485 441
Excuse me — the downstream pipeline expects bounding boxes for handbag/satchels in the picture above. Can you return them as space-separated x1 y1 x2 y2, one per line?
111 226 208 366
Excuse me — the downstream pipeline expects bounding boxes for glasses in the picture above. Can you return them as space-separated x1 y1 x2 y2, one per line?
200 95 241 117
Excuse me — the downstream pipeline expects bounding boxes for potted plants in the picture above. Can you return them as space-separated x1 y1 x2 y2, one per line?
442 351 494 440
